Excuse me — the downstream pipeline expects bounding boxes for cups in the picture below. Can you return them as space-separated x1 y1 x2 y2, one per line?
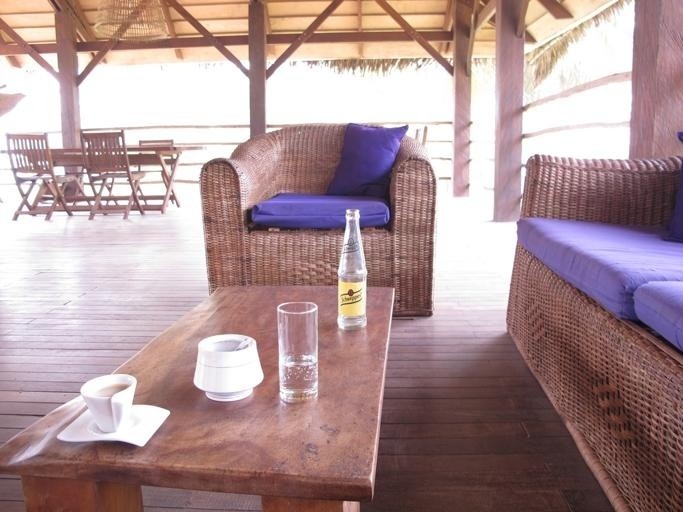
275 300 320 404
80 373 138 434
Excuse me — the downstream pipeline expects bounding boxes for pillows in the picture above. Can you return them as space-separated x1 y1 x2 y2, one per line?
327 124 409 196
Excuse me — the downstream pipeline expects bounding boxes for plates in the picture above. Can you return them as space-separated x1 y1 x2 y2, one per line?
55 402 171 447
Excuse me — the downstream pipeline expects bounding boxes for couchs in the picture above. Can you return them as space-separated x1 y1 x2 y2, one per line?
505 156 682 511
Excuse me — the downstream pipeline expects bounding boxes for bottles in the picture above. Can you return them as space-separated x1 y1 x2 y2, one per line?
336 208 368 331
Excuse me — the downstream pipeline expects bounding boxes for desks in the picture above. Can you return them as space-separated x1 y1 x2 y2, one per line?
0 145 203 213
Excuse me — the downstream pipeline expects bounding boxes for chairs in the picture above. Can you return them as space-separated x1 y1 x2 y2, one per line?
198 124 435 316
5 128 181 221
